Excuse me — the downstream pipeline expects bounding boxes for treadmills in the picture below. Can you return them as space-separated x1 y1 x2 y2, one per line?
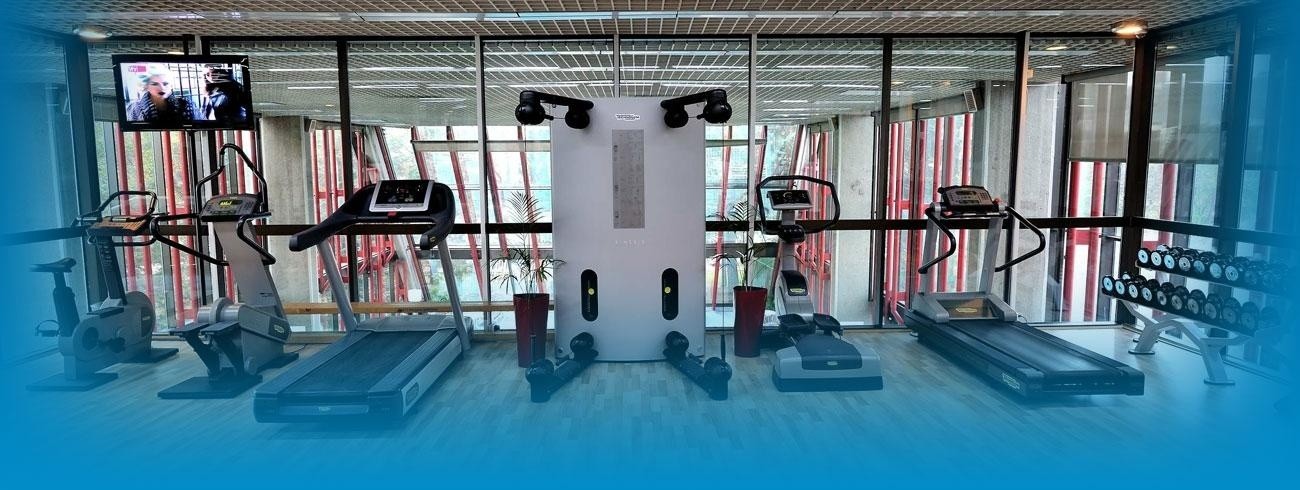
904 185 1144 399
253 180 474 424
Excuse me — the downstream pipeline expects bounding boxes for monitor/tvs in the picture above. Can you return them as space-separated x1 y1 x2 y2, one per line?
110 53 255 132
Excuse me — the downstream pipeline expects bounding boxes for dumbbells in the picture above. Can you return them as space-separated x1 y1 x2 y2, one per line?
1137 244 1299 298
1101 271 1280 333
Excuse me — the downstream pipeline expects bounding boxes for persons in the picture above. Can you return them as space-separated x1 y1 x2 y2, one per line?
126 67 201 122
201 64 247 119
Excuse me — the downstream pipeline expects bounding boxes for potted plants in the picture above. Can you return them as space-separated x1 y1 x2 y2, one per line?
707 198 781 357
479 188 566 370
